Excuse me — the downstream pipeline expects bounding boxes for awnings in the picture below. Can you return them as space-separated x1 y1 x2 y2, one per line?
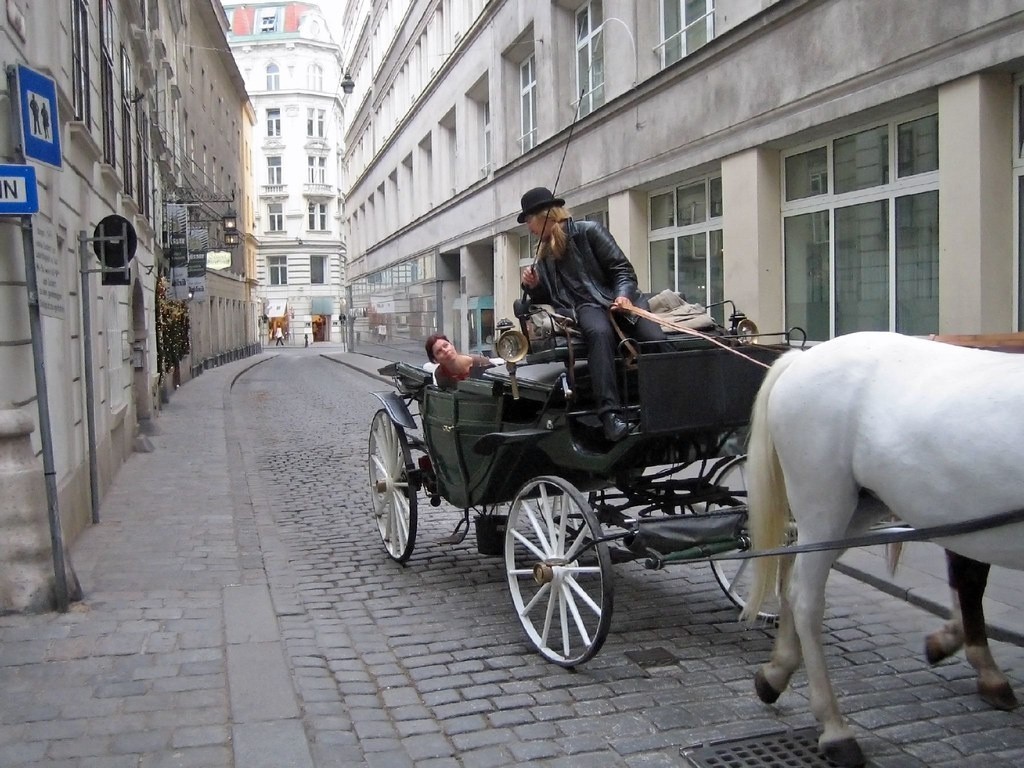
312 297 333 316
266 299 287 317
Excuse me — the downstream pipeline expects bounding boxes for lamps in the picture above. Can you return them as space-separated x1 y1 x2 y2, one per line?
340 68 355 94
298 239 303 247
188 203 239 250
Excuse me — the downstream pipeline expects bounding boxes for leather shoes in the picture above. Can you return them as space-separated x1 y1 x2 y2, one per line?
603 412 635 441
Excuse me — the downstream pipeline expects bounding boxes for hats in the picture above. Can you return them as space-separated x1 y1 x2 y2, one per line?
517 186 565 223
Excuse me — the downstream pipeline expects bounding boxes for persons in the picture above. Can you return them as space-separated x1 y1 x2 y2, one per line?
274 326 285 346
517 186 673 440
425 334 497 390
375 323 386 344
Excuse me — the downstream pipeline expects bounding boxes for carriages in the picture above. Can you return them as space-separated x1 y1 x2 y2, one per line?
367 285 1024 768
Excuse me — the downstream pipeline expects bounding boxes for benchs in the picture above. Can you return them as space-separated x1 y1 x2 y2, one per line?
513 291 738 439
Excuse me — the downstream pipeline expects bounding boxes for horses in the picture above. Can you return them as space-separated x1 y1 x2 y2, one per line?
742 331 1024 768
